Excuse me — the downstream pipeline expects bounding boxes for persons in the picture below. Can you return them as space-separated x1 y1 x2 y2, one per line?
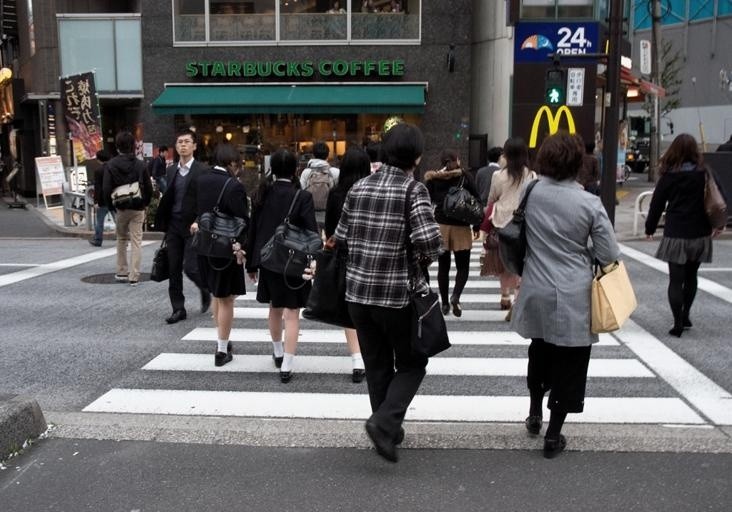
335 122 446 463
102 131 153 286
89 150 118 246
152 131 539 382
717 134 731 153
519 129 619 459
643 134 726 337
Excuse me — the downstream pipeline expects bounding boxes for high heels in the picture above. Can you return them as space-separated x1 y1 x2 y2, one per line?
442 289 464 318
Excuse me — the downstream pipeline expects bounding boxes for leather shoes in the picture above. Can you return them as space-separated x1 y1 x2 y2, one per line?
198 292 212 312
522 414 544 434
272 353 296 383
666 314 694 338
350 366 367 386
542 434 567 459
500 295 512 311
212 342 234 366
164 309 188 324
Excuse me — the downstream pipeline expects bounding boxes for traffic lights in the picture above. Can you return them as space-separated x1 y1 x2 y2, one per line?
546 68 564 105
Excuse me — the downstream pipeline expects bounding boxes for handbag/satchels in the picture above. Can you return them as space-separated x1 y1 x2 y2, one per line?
703 174 729 233
301 243 355 327
498 210 525 277
590 262 638 334
109 182 142 208
434 186 485 227
410 294 454 357
148 247 184 286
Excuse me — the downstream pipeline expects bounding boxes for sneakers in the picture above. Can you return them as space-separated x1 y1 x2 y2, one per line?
114 272 140 287
88 237 103 246
364 417 405 465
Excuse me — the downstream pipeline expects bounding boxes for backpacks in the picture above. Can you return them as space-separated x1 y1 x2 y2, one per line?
147 159 155 178
259 187 320 279
306 168 336 212
194 175 246 273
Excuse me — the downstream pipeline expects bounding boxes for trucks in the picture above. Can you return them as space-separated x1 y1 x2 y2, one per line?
626 111 674 172
